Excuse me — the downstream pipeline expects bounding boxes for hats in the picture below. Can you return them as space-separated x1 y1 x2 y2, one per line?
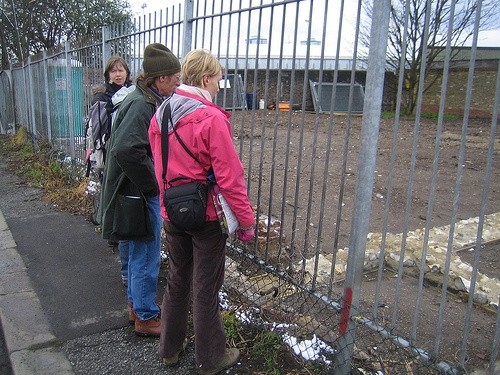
143 43 181 79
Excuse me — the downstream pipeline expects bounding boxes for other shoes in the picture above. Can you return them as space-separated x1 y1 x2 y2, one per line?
108 240 119 247
122 275 128 287
128 302 137 323
135 319 161 337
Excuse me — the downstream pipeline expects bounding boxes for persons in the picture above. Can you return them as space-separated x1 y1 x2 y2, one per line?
147 47 256 375
100 43 181 338
85 55 137 247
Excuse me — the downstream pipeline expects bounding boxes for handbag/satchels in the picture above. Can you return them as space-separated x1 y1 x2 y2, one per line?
163 181 206 231
208 174 240 235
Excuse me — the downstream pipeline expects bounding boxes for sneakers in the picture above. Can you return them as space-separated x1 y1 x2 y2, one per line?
163 338 187 365
198 348 240 375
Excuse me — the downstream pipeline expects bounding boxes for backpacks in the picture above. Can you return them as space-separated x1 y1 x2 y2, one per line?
88 98 123 150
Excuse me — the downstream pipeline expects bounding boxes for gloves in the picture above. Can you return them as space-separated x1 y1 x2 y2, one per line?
237 229 254 241
